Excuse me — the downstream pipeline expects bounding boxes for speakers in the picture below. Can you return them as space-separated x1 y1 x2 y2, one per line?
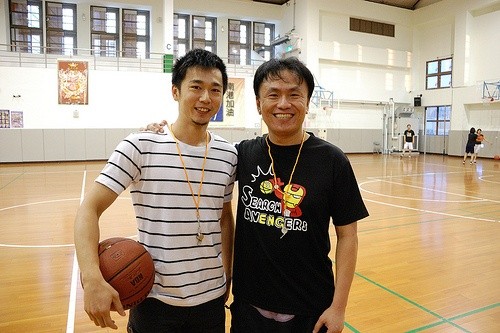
414 97 421 106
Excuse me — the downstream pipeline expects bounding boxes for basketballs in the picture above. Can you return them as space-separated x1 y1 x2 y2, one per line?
80 238 155 312
494 155 499 160
478 136 483 141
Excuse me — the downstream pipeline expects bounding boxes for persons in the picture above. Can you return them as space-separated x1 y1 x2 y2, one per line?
463 127 481 164
400 124 415 158
74 49 239 333
139 56 370 333
473 129 484 163
